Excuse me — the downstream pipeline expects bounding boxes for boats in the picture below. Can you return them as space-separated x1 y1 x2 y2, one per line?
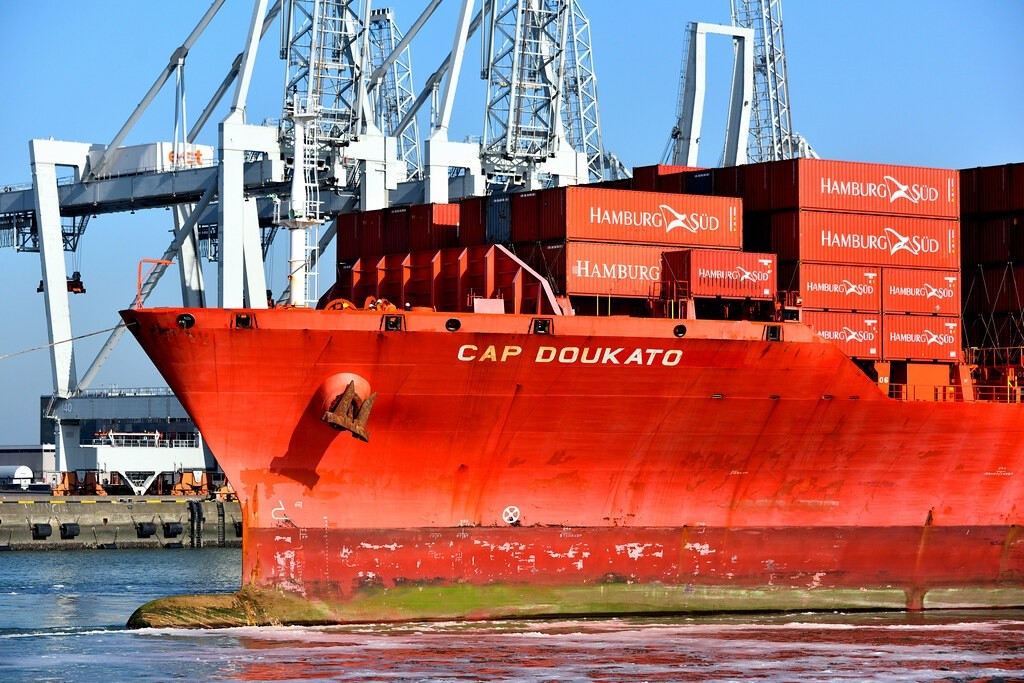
120 1 1024 614
